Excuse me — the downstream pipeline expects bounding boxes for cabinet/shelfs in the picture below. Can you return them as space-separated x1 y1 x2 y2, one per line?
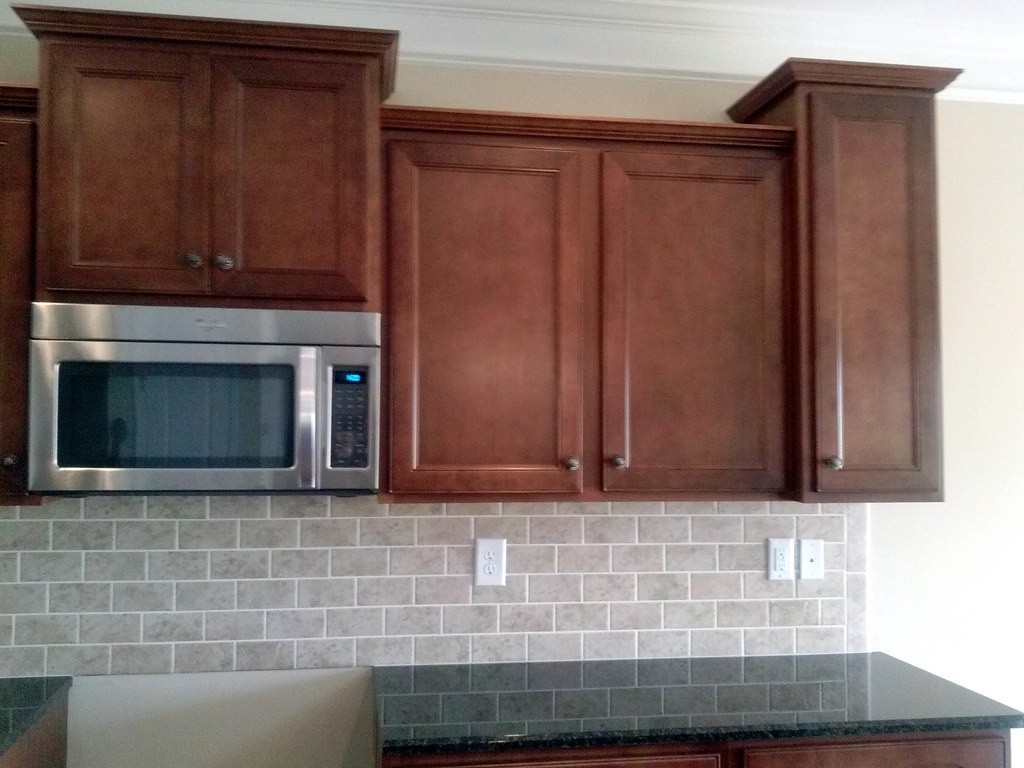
12 2 400 312
375 729 1008 767
0 80 61 506
381 103 798 504
0 695 66 768
725 57 967 503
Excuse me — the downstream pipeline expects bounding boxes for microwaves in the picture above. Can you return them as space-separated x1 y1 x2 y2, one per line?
29 299 380 489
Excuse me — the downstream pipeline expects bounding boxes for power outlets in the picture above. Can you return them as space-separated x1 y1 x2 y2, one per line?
767 536 796 580
475 538 506 586
